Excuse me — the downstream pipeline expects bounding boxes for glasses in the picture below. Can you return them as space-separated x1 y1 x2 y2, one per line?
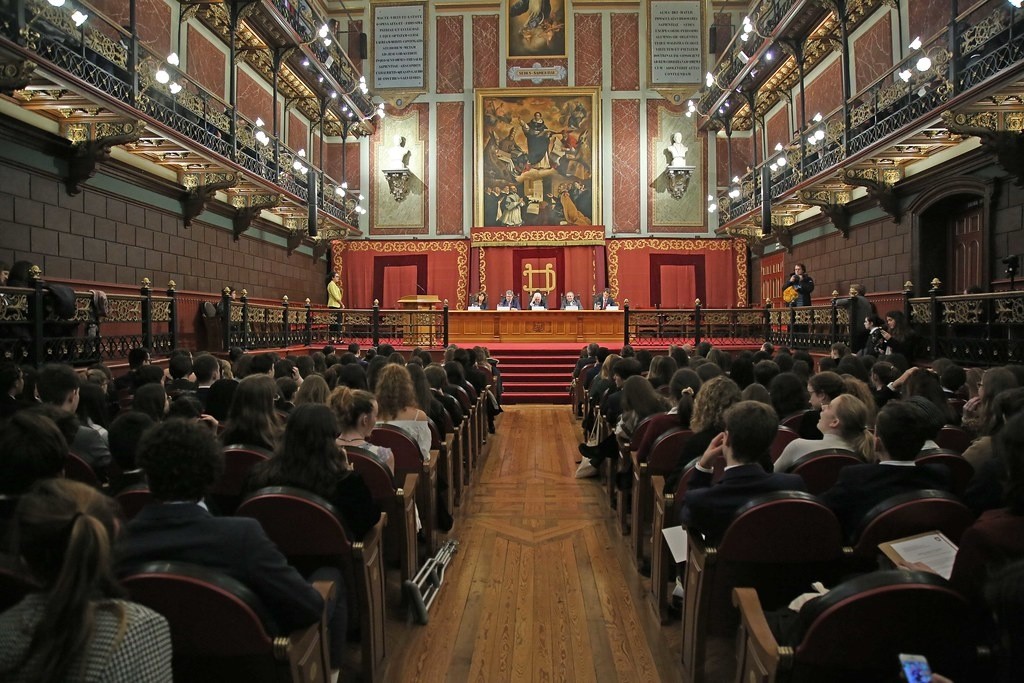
273 393 280 400
166 396 172 401
148 359 152 362
808 391 816 397
976 382 983 390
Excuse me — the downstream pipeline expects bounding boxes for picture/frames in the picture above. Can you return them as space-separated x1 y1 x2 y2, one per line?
473 85 605 228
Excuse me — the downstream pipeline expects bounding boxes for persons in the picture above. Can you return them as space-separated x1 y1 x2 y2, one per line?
527 291 548 310
214 287 237 315
836 283 919 368
472 291 489 310
328 18 340 37
112 416 328 637
1 341 505 534
0 475 175 683
559 291 584 310
0 261 10 287
327 271 347 345
566 340 1024 683
667 132 688 167
499 290 522 310
595 287 615 310
782 263 815 308
792 117 819 167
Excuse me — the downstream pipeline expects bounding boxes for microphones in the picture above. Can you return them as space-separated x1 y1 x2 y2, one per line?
414 283 426 295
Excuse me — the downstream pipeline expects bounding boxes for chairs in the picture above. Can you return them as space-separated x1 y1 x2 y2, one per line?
10 351 1024 683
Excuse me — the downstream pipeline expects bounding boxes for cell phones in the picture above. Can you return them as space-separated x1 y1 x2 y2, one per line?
898 653 931 683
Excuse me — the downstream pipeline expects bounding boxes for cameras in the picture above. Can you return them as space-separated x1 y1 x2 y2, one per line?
872 325 887 339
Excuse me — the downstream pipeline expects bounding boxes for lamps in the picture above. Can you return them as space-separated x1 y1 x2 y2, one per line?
20 0 90 41
341 75 369 97
136 52 184 106
323 181 349 197
769 142 803 177
345 193 368 221
898 36 953 92
299 24 332 49
278 148 308 185
806 111 844 152
728 176 755 202
705 72 732 92
741 17 771 40
707 194 731 218
236 117 270 159
685 100 708 119
362 103 386 121
